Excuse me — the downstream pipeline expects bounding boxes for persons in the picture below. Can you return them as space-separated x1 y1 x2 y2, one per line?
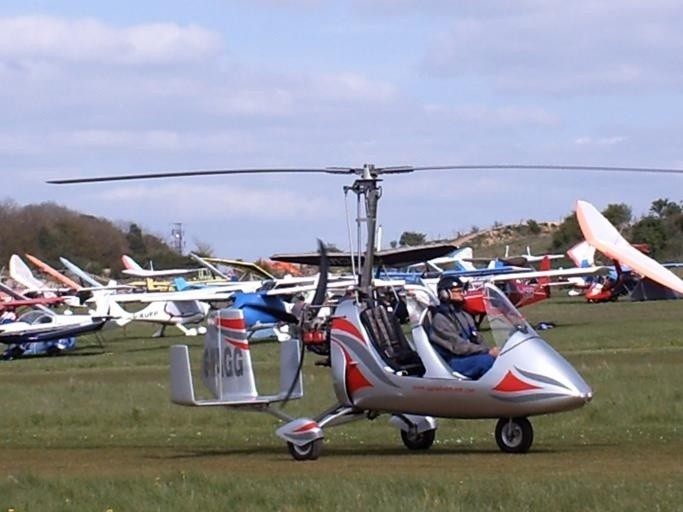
419 275 503 381
288 294 307 318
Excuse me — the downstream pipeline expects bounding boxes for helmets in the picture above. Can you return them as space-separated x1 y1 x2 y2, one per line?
435 276 465 301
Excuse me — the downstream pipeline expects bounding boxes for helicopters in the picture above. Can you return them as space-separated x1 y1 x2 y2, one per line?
46 164 683 461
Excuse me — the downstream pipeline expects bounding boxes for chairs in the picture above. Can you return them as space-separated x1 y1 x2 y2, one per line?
360 303 422 377
